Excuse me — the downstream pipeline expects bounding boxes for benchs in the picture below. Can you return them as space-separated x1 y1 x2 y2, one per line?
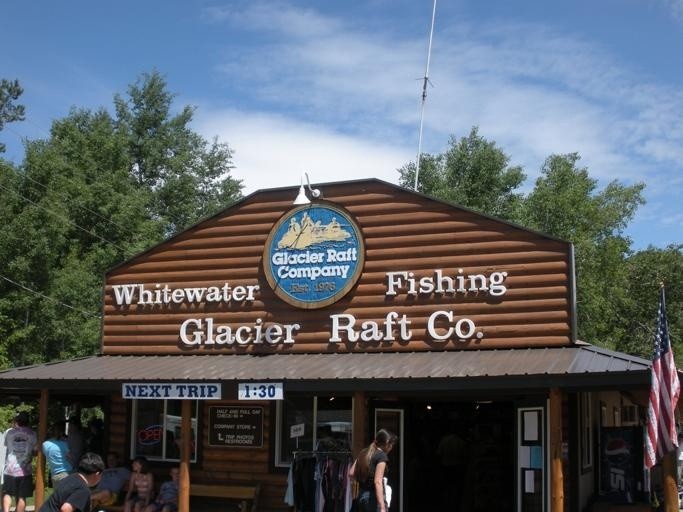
96 480 263 512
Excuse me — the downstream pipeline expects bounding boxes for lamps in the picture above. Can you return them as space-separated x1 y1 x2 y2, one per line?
294 171 320 206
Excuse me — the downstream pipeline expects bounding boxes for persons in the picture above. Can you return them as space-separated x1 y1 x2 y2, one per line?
435 422 465 511
346 428 396 512
0 410 179 511
316 425 336 452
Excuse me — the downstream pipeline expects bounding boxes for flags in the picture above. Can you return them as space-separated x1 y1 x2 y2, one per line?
642 289 681 469
